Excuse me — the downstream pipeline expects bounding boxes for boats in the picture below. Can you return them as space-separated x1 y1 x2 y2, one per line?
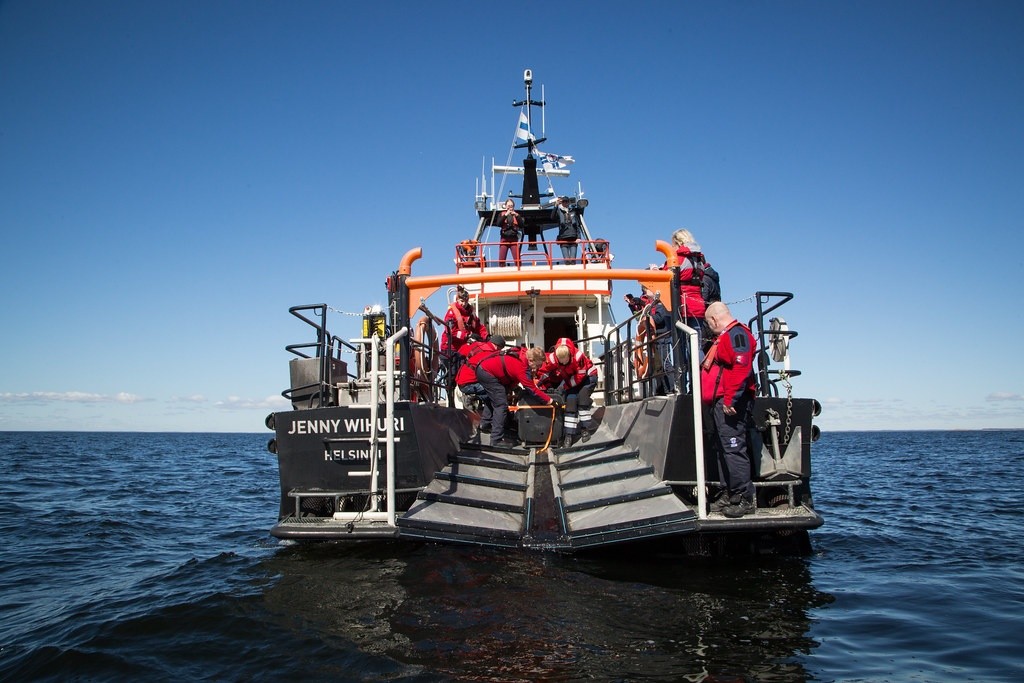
263 71 824 552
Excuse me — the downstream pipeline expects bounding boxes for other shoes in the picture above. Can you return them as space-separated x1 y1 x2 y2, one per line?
582 430 592 443
491 440 513 450
448 399 455 408
564 435 572 450
480 424 492 430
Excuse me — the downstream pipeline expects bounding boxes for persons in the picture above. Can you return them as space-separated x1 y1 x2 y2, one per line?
641 227 720 399
551 196 579 265
700 302 757 515
496 199 523 266
441 289 598 449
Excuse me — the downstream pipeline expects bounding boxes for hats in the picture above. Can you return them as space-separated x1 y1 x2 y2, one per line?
561 196 569 202
489 335 505 349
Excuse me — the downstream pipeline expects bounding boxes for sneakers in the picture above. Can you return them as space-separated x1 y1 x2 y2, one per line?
722 495 755 518
707 491 731 511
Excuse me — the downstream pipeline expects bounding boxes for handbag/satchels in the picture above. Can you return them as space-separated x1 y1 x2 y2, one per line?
732 395 755 422
